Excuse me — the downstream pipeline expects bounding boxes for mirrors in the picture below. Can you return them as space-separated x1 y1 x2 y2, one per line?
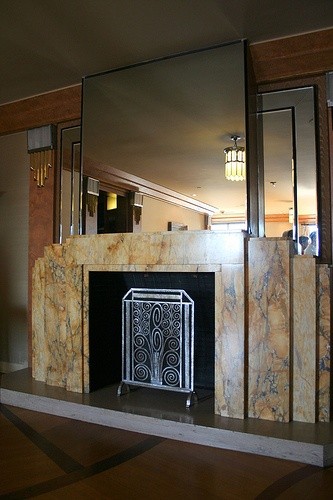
56 39 319 261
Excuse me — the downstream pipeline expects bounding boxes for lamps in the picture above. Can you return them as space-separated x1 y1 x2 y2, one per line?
223 136 245 182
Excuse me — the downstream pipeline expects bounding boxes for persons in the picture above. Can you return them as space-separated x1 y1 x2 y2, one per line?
298 236 310 256
282 229 298 256
304 231 317 256
